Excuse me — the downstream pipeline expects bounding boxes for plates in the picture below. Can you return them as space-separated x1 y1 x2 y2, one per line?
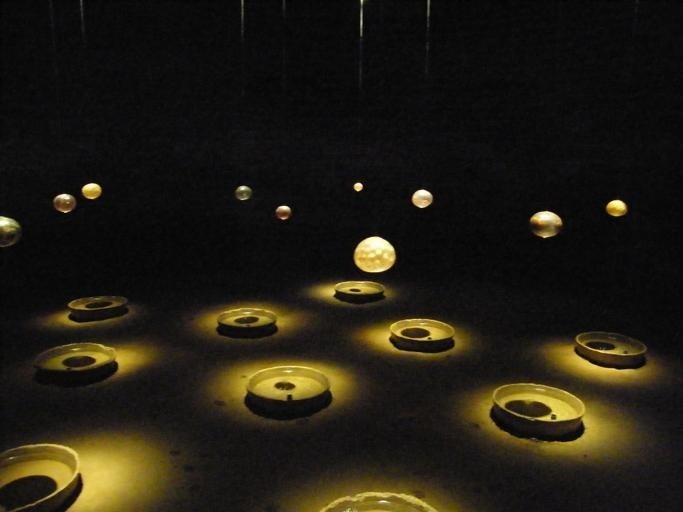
335 279 388 303
217 308 277 338
492 382 586 432
246 366 331 405
31 342 117 381
574 330 648 367
0 443 79 511
69 295 129 317
387 318 456 351
